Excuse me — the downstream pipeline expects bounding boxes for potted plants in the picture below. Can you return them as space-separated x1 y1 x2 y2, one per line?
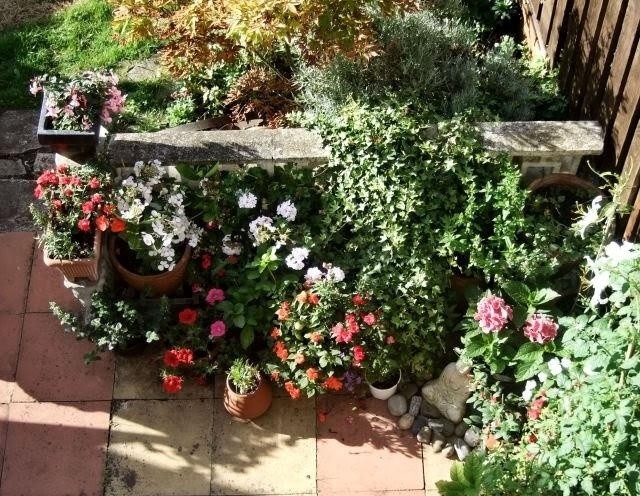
224 359 271 419
366 325 401 400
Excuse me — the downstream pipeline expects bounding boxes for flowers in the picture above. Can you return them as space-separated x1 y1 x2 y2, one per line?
445 159 640 496
29 154 385 398
29 69 125 132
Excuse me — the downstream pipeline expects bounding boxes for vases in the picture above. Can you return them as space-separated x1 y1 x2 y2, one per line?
108 234 191 294
43 223 102 283
